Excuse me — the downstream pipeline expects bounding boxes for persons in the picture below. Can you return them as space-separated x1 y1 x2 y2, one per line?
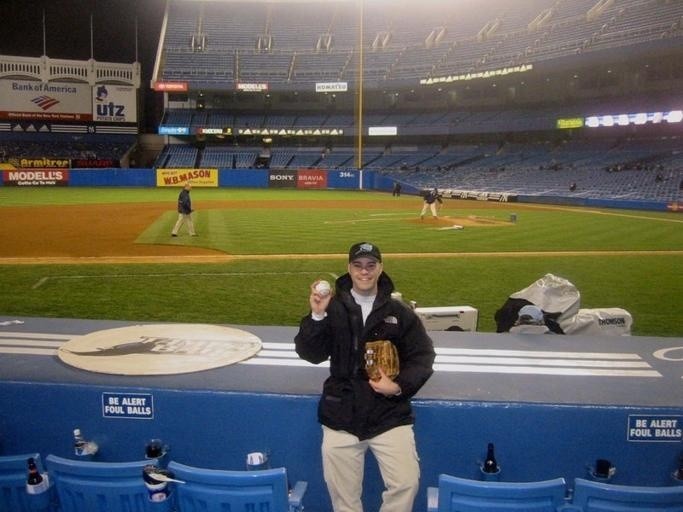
171 183 198 237
419 188 443 220
606 162 663 174
507 304 556 335
654 173 663 187
568 182 576 193
391 181 400 196
0 139 132 160
292 242 436 512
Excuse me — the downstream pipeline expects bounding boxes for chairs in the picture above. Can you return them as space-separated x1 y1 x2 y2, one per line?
572 477 681 510
46 454 160 511
426 474 568 510
0 138 137 163
1 454 54 511
166 461 308 510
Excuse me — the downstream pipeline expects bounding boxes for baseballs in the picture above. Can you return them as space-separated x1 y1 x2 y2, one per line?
315 280 330 296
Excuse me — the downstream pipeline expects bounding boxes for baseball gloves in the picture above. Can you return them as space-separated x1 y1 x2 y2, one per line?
365 341 399 382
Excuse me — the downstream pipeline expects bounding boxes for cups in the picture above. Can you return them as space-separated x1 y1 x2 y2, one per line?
144 481 169 502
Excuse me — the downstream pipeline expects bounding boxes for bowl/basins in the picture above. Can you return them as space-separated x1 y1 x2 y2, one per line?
142 464 163 484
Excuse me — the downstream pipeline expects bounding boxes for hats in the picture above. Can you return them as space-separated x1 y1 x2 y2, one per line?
347 241 382 264
517 303 543 324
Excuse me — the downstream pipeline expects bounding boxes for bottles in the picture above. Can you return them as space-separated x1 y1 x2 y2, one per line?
72 428 84 454
145 432 163 458
26 457 42 484
484 441 498 474
592 459 610 478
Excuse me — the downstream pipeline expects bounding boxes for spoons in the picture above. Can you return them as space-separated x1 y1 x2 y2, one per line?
148 473 185 484
